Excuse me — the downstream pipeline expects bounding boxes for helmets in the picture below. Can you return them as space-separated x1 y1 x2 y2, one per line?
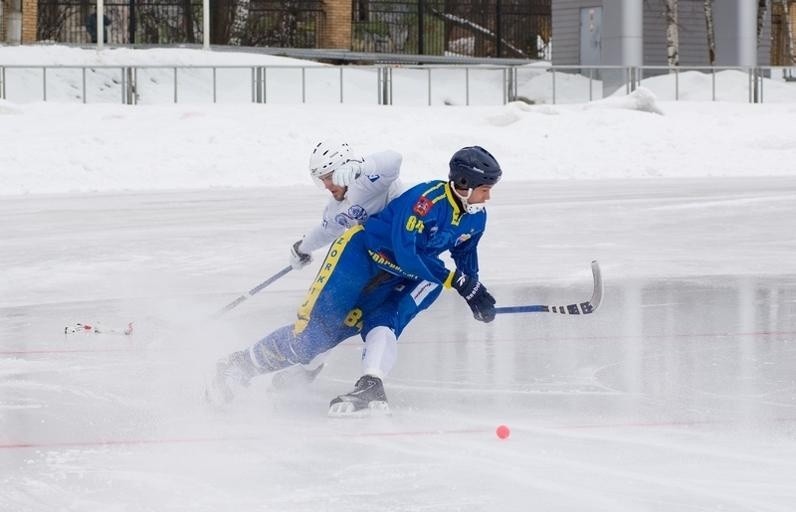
449 146 502 188
310 135 363 176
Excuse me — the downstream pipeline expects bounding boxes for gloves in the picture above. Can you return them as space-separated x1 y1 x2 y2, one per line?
451 269 495 322
289 241 310 270
332 164 361 186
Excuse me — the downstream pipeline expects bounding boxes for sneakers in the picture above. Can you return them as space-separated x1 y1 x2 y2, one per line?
330 376 387 410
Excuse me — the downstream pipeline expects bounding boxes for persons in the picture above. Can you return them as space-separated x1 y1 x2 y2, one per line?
217 147 501 412
86 6 110 43
272 140 442 408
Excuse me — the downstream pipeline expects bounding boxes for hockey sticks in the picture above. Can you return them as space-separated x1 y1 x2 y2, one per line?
143 256 309 329
495 261 601 314
